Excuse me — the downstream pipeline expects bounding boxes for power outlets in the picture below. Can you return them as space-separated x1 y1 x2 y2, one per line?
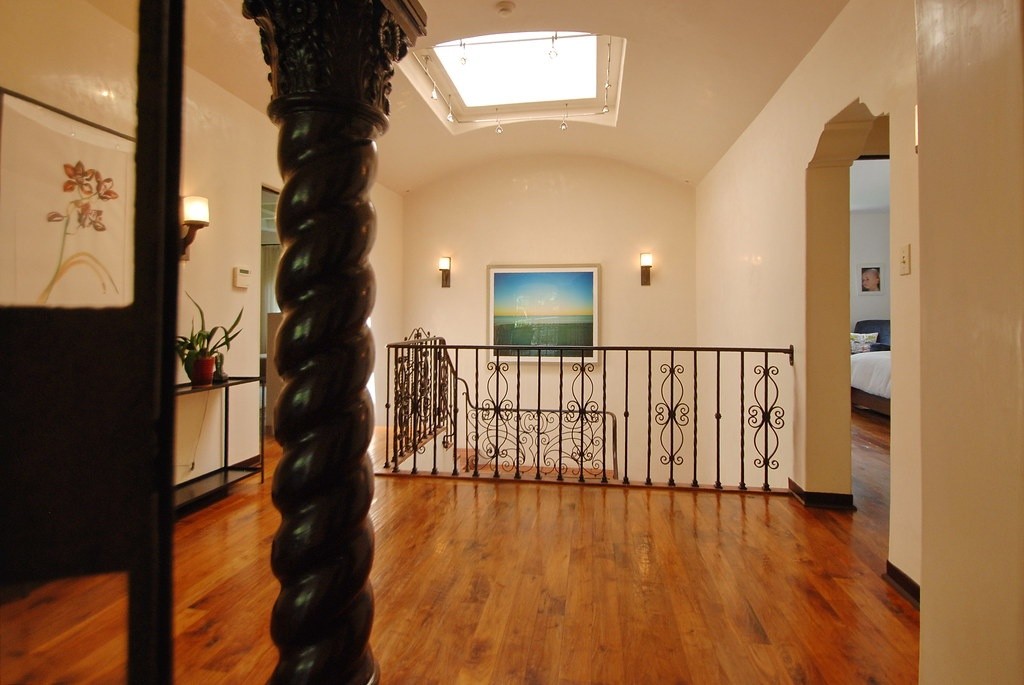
899 243 913 276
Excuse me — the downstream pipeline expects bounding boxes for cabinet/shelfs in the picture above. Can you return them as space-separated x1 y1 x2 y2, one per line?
173 376 265 511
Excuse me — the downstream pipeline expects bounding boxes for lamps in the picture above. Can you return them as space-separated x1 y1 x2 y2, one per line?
446 103 455 123
603 99 610 113
639 252 653 286
179 195 211 260
605 81 612 88
546 37 559 59
422 56 432 75
431 81 438 102
458 44 469 65
438 256 452 288
496 119 504 136
558 114 569 132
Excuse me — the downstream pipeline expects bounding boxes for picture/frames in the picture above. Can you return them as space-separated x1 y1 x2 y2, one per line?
857 264 884 295
486 264 602 368
0 86 133 308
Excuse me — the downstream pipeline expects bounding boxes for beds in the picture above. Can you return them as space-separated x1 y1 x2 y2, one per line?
851 320 891 417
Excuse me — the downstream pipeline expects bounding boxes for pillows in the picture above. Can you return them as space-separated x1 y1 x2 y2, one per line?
850 333 878 353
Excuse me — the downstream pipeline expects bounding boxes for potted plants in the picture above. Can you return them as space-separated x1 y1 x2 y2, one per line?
173 291 243 388
